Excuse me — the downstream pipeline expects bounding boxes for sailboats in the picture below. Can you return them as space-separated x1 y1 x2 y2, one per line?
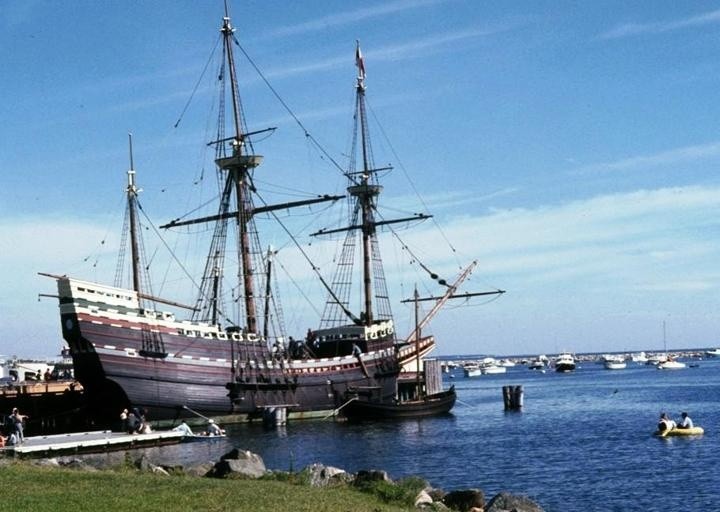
32 3 509 429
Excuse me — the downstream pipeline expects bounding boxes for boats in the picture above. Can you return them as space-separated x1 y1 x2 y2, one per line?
0 345 86 413
430 349 720 382
0 404 248 479
652 426 705 438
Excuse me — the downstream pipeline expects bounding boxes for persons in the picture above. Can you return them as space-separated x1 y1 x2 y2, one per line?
676 412 694 429
35 367 75 387
0 405 30 449
170 419 194 436
351 343 363 355
119 406 149 435
657 412 677 431
271 326 318 360
201 418 223 437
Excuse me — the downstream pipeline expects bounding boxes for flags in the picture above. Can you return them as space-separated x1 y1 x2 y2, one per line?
354 39 367 79
